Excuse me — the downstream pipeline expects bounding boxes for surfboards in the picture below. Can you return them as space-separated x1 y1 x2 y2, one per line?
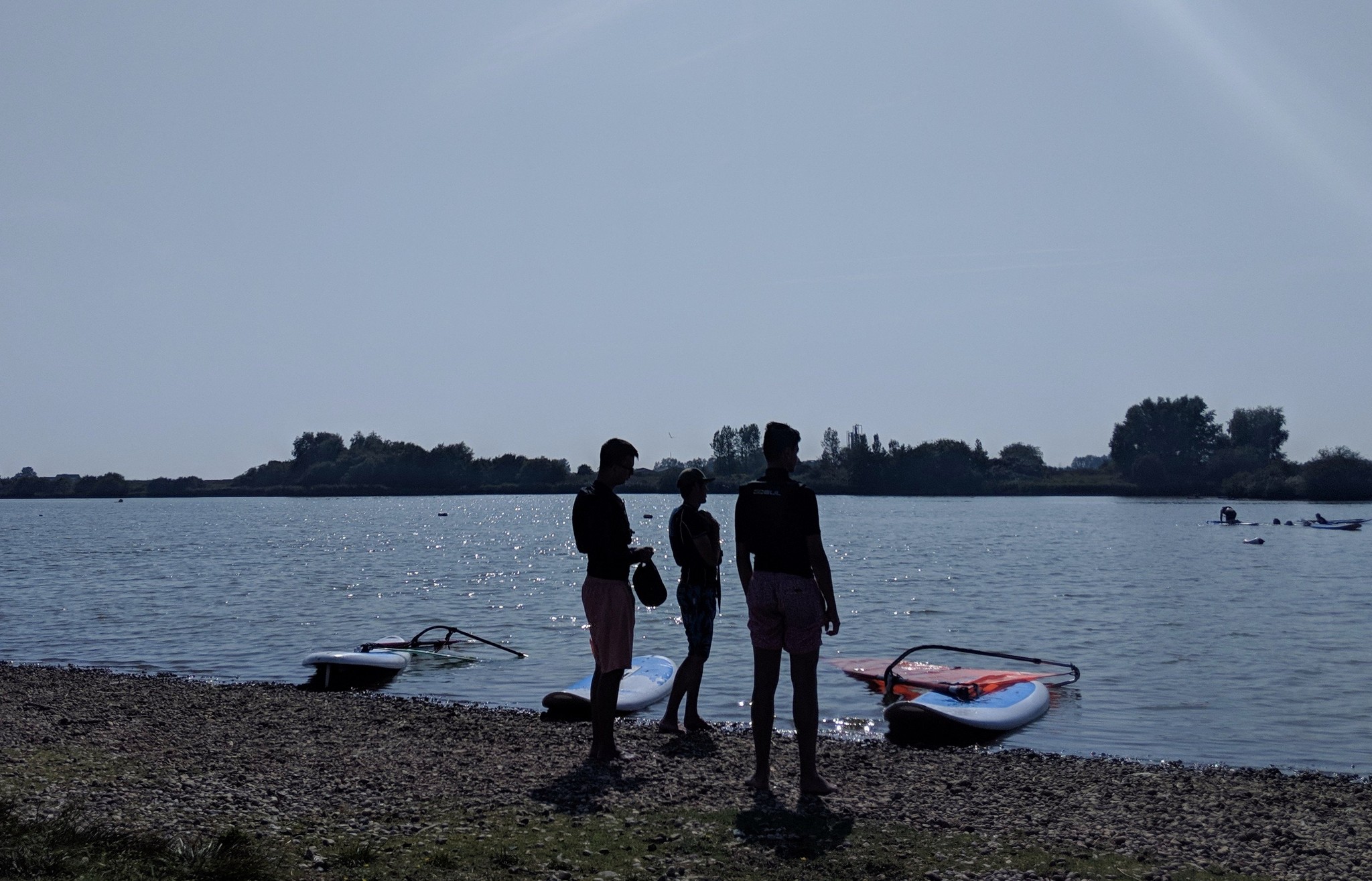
883 679 1052 729
540 654 678 714
299 635 412 672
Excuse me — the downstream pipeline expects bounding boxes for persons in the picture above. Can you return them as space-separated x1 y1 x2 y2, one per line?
734 422 842 796
571 439 654 763
657 467 724 734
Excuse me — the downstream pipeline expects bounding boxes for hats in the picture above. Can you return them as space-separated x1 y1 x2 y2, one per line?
676 468 715 488
633 559 667 607
